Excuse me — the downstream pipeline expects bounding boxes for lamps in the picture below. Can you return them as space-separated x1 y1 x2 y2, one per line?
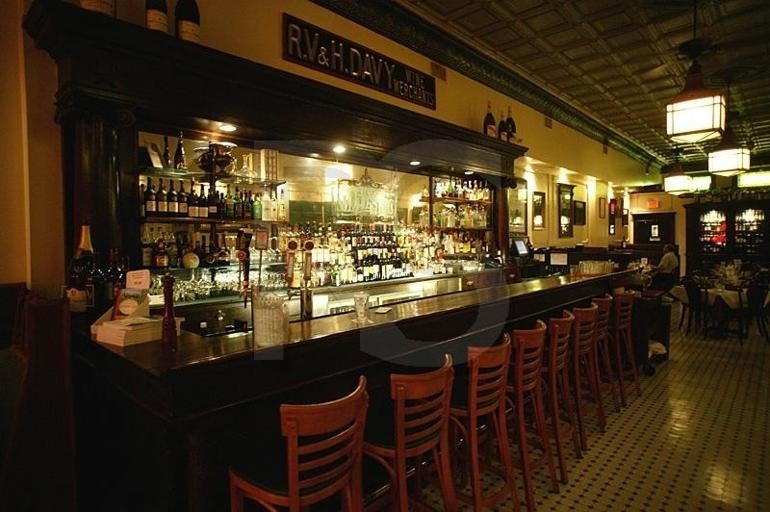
662 0 751 195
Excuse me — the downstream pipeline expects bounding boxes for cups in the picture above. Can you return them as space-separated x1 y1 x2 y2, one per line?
568 258 615 276
353 294 369 321
641 257 648 268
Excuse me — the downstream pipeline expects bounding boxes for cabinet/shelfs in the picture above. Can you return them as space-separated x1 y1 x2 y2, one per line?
428 176 493 232
141 168 285 272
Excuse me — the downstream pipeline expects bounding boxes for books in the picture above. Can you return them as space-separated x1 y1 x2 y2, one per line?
96 315 186 347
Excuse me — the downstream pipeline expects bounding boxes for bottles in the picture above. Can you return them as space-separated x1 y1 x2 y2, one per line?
68 225 129 314
77 1 202 42
140 130 496 305
483 100 517 146
621 235 627 248
160 277 178 347
699 210 766 254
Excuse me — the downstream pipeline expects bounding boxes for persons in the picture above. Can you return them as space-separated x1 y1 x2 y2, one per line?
653 243 679 273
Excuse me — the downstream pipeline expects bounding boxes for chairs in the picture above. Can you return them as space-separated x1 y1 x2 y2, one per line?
640 261 770 346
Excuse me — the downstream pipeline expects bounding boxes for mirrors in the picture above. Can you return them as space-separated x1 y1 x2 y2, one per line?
508 179 527 238
533 192 546 231
558 182 576 239
